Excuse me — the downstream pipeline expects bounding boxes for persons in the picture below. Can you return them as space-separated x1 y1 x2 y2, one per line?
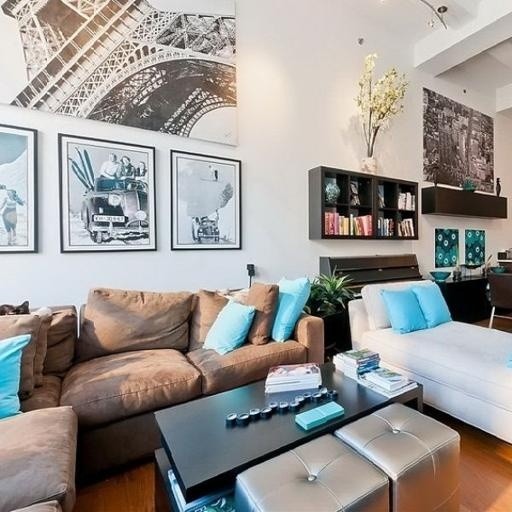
0 190 24 246
98 153 148 181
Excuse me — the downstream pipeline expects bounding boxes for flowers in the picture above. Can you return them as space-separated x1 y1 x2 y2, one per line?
356 49 408 158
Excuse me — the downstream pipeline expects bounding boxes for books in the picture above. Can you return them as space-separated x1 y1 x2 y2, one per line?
265 363 322 393
325 192 415 236
333 347 416 398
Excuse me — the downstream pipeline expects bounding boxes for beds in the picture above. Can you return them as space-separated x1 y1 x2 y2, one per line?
319 258 512 445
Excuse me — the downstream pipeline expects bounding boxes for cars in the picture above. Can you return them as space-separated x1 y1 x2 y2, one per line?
195 219 220 243
80 177 149 244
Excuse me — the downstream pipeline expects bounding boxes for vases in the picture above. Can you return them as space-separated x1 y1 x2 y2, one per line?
361 157 377 175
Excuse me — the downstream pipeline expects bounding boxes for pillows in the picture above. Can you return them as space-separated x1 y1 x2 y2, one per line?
0 333 31 419
203 298 258 355
360 279 434 328
384 289 425 333
272 277 312 342
410 284 452 328
245 283 278 344
25 307 54 386
0 315 38 399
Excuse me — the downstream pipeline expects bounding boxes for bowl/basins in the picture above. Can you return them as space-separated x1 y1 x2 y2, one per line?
490 267 507 273
430 272 451 282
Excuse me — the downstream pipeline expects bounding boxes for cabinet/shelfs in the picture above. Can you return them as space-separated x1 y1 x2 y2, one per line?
422 186 507 220
309 165 420 241
435 276 512 324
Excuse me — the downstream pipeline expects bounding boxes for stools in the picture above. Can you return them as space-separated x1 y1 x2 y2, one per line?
335 400 462 511
234 432 390 511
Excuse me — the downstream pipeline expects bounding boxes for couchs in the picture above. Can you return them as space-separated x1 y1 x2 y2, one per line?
0 289 329 512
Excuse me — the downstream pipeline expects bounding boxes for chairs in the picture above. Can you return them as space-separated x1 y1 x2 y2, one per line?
486 272 512 330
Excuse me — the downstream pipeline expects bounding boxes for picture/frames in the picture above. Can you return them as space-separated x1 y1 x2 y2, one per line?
169 148 242 254
58 128 158 255
0 123 41 254
